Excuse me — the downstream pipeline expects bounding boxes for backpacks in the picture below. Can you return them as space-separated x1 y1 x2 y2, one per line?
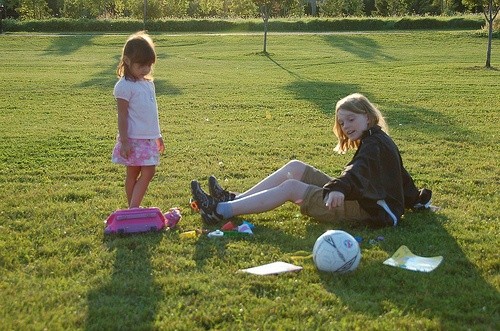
106 206 180 233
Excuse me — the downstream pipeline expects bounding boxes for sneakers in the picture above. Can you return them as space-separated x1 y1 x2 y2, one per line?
207 175 236 203
191 180 222 226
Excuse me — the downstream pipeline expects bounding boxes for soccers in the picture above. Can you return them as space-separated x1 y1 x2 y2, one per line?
313 230 362 273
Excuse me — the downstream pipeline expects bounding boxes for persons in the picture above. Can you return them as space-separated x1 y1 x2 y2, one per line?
112 31 165 209
191 93 432 228
